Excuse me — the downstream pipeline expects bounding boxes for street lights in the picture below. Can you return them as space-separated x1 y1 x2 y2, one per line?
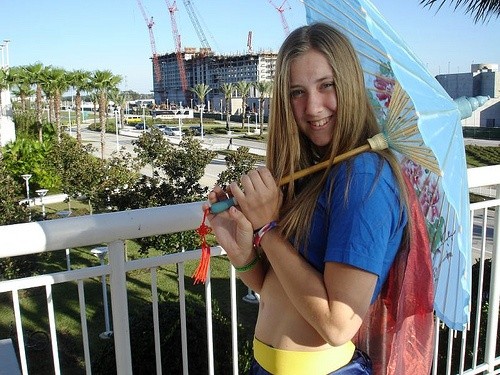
35 189 49 221
90 246 114 340
0 39 11 69
255 113 258 129
21 174 32 222
56 211 72 271
247 116 251 133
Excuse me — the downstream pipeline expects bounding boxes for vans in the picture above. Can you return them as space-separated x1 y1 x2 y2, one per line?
165 127 182 136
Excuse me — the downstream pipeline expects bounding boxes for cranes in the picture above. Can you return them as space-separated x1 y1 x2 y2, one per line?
164 0 193 107
270 1 291 37
137 0 166 101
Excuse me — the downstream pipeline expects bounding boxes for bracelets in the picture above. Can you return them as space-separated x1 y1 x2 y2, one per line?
233 256 260 272
254 221 281 249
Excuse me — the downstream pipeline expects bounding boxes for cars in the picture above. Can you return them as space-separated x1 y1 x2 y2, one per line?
190 126 205 136
155 124 166 132
135 123 148 130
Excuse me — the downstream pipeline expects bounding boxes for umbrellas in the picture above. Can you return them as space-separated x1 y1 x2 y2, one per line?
209 0 491 338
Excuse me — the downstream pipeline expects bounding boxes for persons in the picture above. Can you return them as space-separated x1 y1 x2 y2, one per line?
201 22 408 375
